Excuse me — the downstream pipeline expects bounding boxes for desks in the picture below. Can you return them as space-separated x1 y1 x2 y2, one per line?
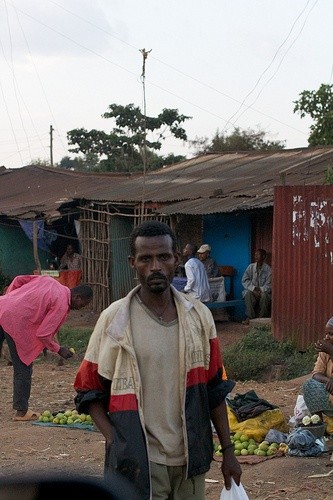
172 277 227 323
32 269 81 289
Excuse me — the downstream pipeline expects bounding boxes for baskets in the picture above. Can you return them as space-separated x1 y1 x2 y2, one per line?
294 423 326 439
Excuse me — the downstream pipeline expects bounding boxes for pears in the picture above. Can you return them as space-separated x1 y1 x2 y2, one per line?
39 410 93 424
69 348 75 353
212 430 279 456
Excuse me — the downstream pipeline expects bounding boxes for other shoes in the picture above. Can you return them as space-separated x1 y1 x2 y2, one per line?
242 319 250 325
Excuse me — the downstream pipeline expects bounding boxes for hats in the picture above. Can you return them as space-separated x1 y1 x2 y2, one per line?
198 244 211 253
182 243 193 257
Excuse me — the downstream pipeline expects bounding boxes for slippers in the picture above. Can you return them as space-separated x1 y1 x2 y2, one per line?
13 409 43 421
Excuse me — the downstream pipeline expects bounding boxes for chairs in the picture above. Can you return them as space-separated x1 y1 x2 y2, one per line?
219 265 234 314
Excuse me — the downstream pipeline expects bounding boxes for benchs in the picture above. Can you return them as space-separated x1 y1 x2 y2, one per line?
204 300 246 323
